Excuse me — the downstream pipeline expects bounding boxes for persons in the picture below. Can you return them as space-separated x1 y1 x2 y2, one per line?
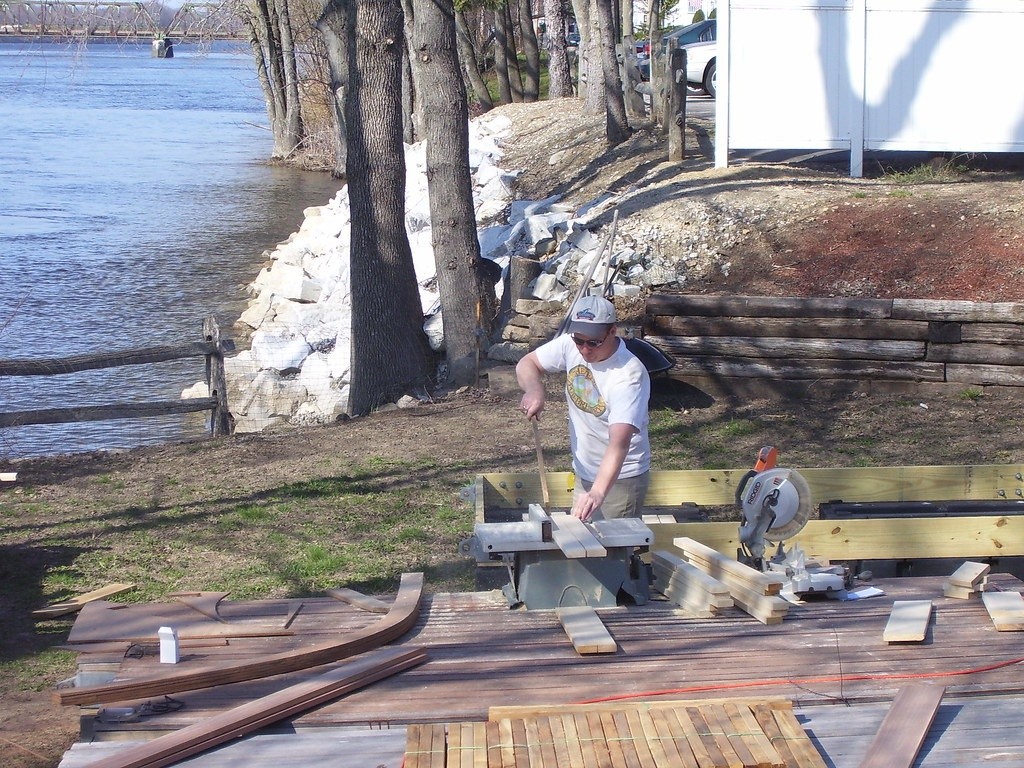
516 295 650 524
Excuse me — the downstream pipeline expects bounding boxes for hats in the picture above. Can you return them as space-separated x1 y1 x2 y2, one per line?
567 295 616 341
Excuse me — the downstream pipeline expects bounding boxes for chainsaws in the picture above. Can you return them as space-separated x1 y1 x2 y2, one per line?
735 446 872 594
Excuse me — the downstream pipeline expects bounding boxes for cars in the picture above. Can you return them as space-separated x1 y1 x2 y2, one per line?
567 32 580 48
620 37 649 67
680 39 716 99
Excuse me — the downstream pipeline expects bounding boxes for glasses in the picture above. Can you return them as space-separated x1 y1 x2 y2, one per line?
571 333 609 348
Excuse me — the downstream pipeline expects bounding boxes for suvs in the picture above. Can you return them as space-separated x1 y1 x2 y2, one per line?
639 18 717 79
